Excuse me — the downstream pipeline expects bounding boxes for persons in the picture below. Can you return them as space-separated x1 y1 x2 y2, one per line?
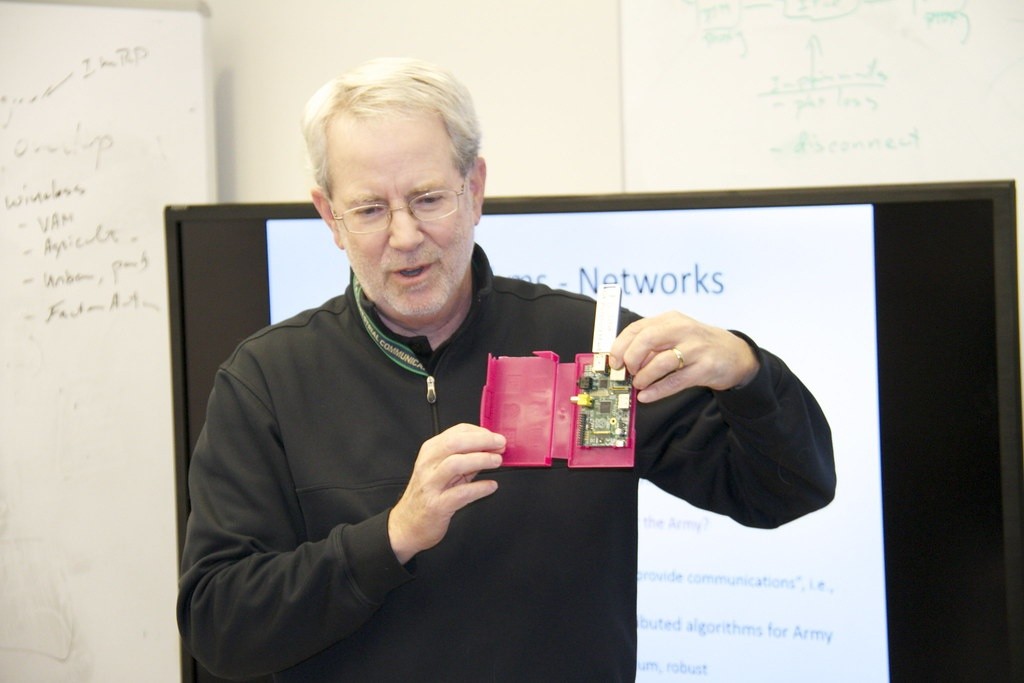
177 56 837 681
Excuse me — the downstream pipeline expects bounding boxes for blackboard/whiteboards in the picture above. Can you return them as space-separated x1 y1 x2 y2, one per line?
2 1 226 683
166 181 1024 683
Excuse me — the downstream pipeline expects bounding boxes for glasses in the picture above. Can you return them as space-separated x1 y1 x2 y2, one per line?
330 174 466 233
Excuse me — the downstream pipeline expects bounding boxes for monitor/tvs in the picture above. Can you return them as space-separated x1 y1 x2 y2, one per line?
165 180 1024 683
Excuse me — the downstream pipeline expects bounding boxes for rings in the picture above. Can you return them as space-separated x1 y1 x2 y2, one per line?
667 347 683 371
